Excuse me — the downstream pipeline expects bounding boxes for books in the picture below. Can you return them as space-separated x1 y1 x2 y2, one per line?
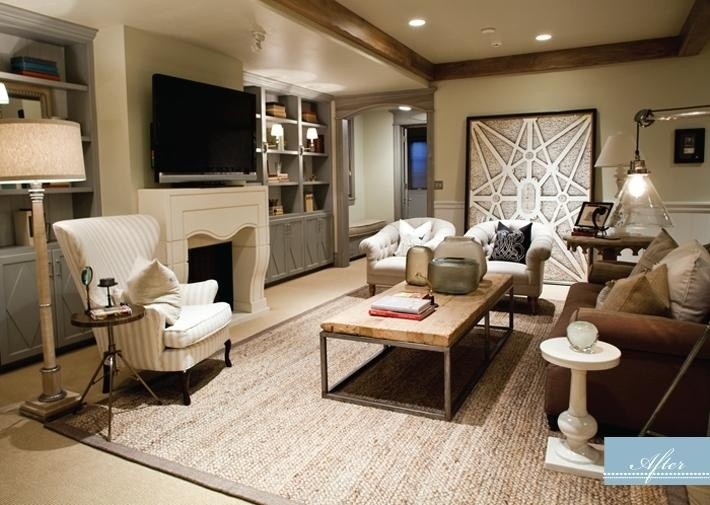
368 293 435 319
88 306 133 320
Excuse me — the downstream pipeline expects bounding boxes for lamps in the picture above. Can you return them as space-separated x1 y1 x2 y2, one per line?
0 118 87 423
604 105 710 228
251 26 266 49
299 127 319 155
263 124 283 153
594 135 647 237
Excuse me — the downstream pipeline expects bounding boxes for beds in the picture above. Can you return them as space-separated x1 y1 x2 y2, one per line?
71 304 163 443
319 273 514 422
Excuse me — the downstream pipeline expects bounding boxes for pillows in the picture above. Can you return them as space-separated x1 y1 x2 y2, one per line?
393 218 432 257
125 256 184 325
489 221 533 264
595 228 710 322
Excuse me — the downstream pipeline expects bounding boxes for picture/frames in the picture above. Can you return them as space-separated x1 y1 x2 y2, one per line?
574 202 614 229
674 128 705 164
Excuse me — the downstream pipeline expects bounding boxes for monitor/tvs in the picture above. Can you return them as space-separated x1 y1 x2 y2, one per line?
150 74 257 184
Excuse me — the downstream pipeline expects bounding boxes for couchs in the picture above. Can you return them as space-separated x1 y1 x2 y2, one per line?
543 244 710 437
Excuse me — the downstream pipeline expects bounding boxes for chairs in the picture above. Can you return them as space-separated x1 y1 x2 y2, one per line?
52 214 232 405
359 217 456 296
464 219 553 309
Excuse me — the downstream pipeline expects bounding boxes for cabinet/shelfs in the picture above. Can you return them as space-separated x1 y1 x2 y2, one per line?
243 70 335 285
0 1 102 367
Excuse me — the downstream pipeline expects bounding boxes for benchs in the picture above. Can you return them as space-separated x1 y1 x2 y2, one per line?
348 220 387 259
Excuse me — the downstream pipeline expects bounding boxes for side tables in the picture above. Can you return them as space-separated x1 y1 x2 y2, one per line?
539 336 622 480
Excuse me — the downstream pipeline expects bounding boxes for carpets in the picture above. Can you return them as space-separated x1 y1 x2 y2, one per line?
42 285 710 505
